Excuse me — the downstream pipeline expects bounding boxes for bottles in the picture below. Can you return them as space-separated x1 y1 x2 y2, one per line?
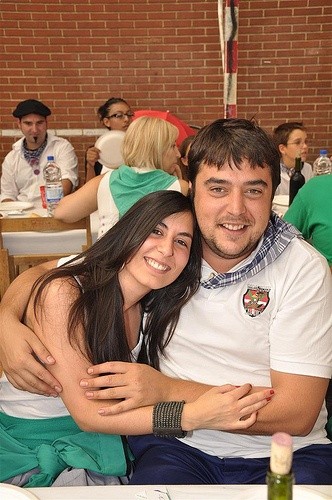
289 150 305 207
43 156 65 217
313 150 332 176
266 431 294 500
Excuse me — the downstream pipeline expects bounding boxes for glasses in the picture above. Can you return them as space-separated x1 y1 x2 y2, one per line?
286 138 311 147
106 111 134 119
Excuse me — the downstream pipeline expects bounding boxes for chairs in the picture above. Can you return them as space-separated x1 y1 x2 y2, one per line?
0 212 92 301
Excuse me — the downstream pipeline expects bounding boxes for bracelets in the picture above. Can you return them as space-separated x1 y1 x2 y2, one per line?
151 401 186 439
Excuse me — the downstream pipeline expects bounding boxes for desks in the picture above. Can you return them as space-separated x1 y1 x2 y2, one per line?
0 200 99 256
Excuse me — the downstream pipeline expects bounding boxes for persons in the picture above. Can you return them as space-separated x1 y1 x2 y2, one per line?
0 189 275 488
0 118 332 485
0 97 332 269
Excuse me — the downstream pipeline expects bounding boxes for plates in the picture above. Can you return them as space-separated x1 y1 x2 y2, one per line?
95 130 126 169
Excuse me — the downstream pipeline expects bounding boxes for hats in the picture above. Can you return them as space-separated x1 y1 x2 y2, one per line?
12 99 50 117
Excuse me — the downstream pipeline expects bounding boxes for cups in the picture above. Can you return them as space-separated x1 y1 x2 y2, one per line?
40 186 47 209
